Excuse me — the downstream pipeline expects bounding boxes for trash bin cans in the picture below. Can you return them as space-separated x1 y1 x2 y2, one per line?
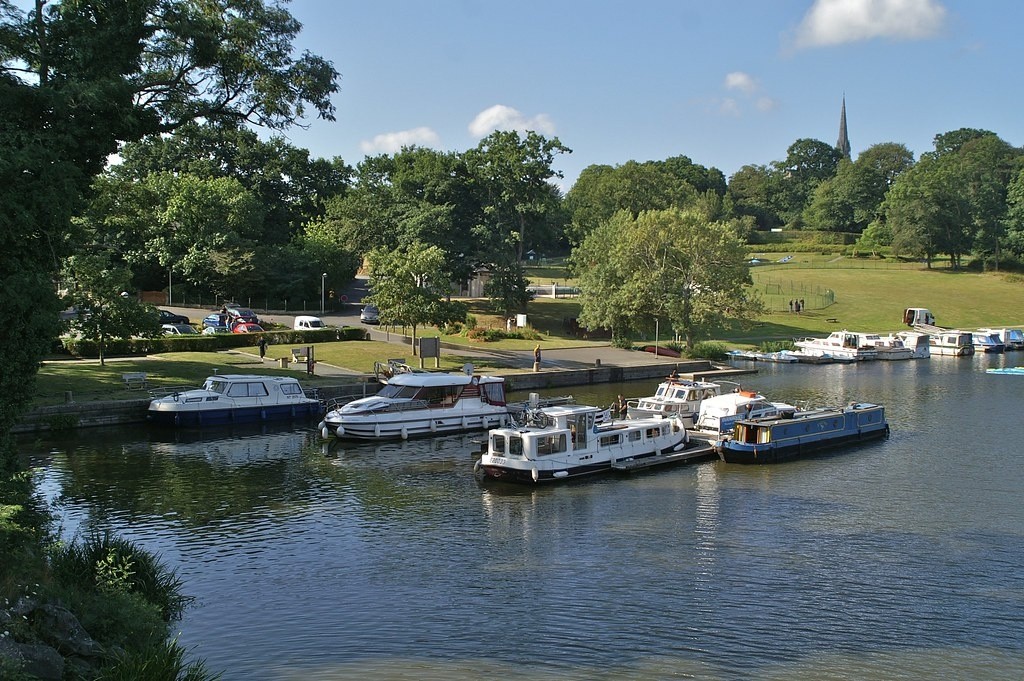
279 357 289 368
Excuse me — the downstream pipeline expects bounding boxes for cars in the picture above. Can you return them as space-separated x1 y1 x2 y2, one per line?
162 324 199 336
224 302 259 324
360 305 381 324
158 310 190 325
233 323 264 334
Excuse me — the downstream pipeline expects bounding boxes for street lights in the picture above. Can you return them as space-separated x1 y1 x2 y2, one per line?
320 272 327 315
99 292 129 365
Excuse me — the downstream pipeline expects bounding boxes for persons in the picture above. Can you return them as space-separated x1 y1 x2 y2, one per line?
225 312 232 330
795 301 801 318
788 299 793 312
506 316 516 332
257 336 268 363
219 310 227 326
795 298 800 308
220 306 227 315
854 335 857 346
845 336 847 342
616 394 628 420
532 344 542 368
799 297 805 311
669 366 681 383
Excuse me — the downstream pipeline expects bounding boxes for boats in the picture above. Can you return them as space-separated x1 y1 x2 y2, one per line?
476 391 716 490
626 370 744 429
686 390 795 437
972 331 1004 353
505 395 576 417
1004 330 1024 350
726 350 834 364
147 374 327 436
896 331 929 358
931 331 974 355
319 371 506 455
862 334 912 361
792 329 878 362
715 402 890 462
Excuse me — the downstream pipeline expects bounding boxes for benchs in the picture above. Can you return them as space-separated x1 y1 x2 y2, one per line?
755 322 765 327
122 373 151 391
824 318 837 323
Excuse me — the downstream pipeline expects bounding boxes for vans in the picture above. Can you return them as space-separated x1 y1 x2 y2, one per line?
202 314 226 331
294 316 328 331
902 308 935 327
